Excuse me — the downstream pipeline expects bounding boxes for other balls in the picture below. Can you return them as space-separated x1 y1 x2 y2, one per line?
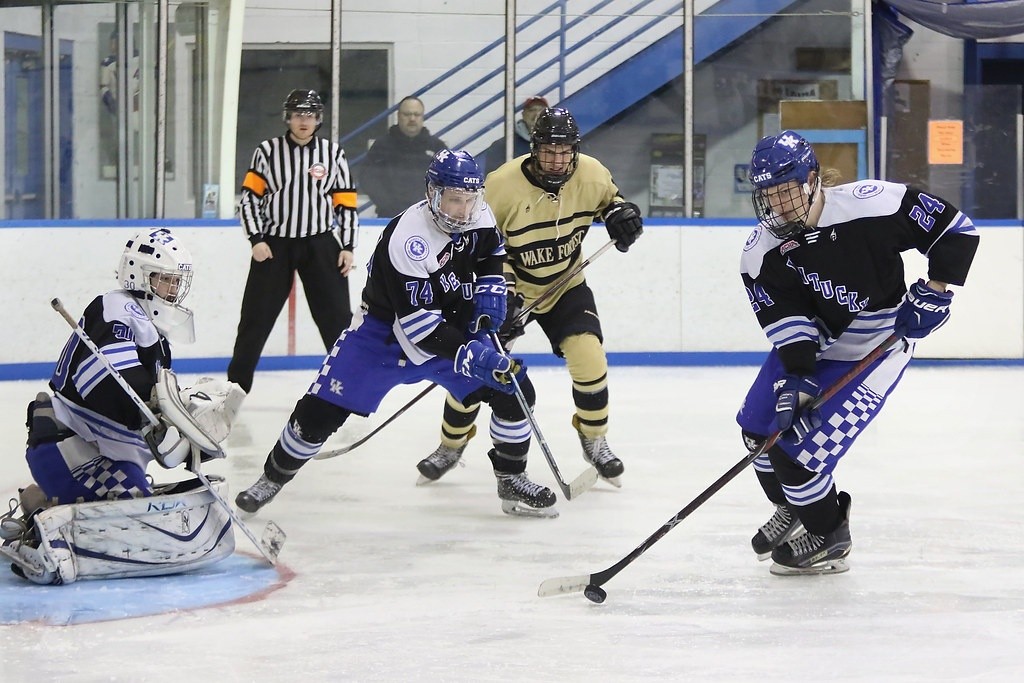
583 585 607 603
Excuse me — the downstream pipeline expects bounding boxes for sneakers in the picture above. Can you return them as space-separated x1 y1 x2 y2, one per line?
487 448 559 518
750 501 805 560
235 473 282 521
571 413 625 489
416 424 477 485
771 491 854 575
0 541 57 585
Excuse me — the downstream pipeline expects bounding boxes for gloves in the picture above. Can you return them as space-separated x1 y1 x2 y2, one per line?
773 374 822 446
468 275 507 334
601 200 643 253
894 279 954 338
495 291 526 345
453 340 527 394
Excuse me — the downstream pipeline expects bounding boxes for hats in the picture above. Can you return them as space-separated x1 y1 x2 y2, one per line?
523 96 548 114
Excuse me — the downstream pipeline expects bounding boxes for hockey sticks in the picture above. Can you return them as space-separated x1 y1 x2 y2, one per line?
481 316 601 502
50 297 287 567
534 331 903 601
311 236 620 462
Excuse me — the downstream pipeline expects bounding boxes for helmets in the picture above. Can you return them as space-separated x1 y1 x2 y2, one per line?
118 227 195 345
282 88 325 114
743 130 822 237
529 109 582 183
424 149 486 235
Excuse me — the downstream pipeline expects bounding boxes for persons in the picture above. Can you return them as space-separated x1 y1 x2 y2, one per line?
736 130 980 575
0 229 246 587
415 109 644 488
226 90 359 394
361 96 445 218
235 146 559 520
485 95 549 178
